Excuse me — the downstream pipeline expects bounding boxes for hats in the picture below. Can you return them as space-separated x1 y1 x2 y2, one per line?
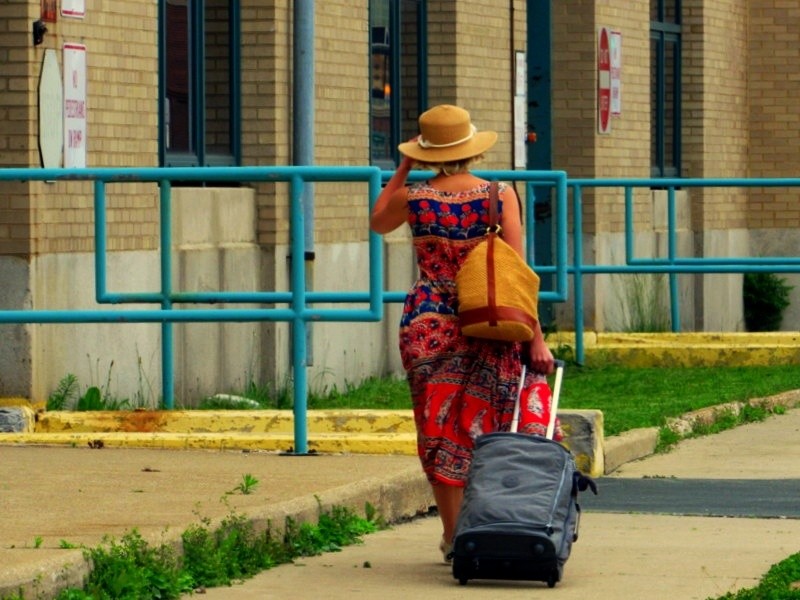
398 104 498 163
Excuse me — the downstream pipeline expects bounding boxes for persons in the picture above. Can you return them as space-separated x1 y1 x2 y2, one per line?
369 104 555 565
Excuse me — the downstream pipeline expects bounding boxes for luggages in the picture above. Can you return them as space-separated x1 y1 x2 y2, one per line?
446 355 599 588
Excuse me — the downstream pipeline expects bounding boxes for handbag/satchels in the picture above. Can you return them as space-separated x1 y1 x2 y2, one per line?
454 233 541 342
